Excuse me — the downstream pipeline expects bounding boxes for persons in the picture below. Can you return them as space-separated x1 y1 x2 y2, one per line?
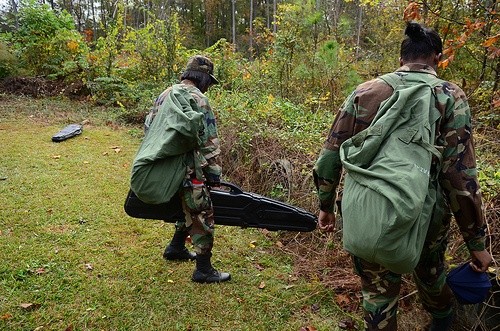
131 54 231 283
311 21 492 331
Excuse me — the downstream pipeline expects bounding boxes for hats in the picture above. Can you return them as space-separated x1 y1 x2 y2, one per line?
186 54 220 85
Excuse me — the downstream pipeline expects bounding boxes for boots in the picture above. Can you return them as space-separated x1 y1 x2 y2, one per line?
164 230 196 260
190 254 231 283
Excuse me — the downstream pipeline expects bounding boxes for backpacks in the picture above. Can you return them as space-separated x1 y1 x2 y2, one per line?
338 83 442 274
129 85 204 205
